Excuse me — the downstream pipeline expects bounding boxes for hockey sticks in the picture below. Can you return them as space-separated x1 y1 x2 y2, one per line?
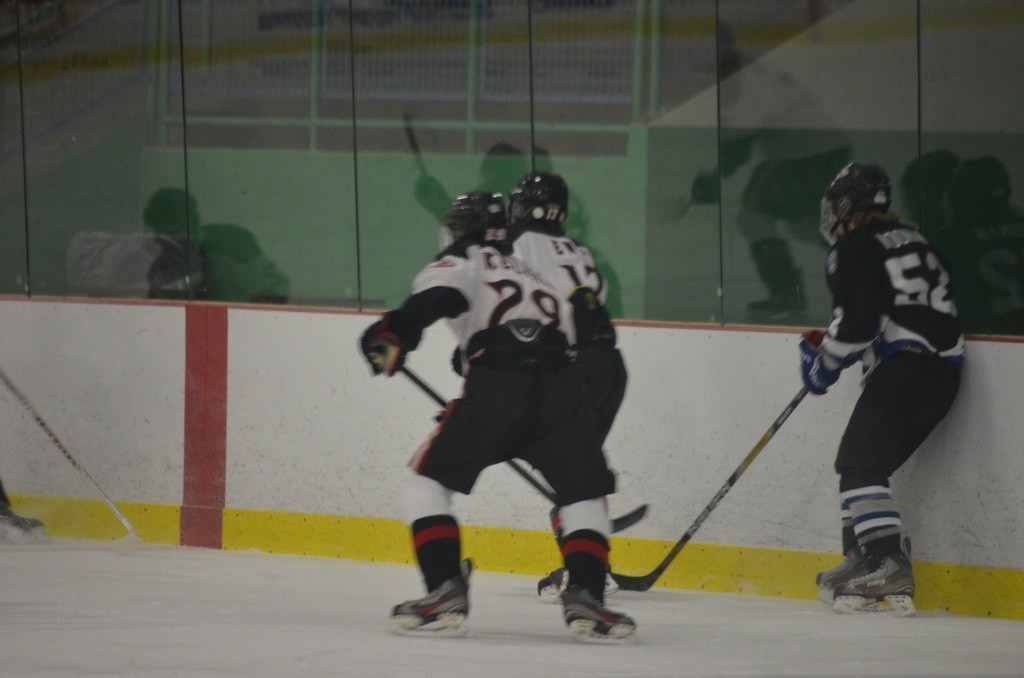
608 382 811 594
371 342 651 536
0 367 146 546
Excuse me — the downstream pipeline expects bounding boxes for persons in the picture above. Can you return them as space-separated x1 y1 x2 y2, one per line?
672 50 850 322
139 186 294 305
796 160 963 615
505 175 628 601
360 192 636 641
414 139 624 320
906 143 1023 337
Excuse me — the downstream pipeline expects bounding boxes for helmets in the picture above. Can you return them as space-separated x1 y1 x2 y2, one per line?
506 172 569 224
440 192 510 245
820 162 891 243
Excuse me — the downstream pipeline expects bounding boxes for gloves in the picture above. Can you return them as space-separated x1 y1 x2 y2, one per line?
799 330 828 358
361 309 422 377
801 352 843 395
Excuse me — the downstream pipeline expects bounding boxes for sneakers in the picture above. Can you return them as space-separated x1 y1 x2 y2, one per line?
538 564 619 598
833 536 917 617
817 544 860 602
391 560 473 632
552 568 635 642
